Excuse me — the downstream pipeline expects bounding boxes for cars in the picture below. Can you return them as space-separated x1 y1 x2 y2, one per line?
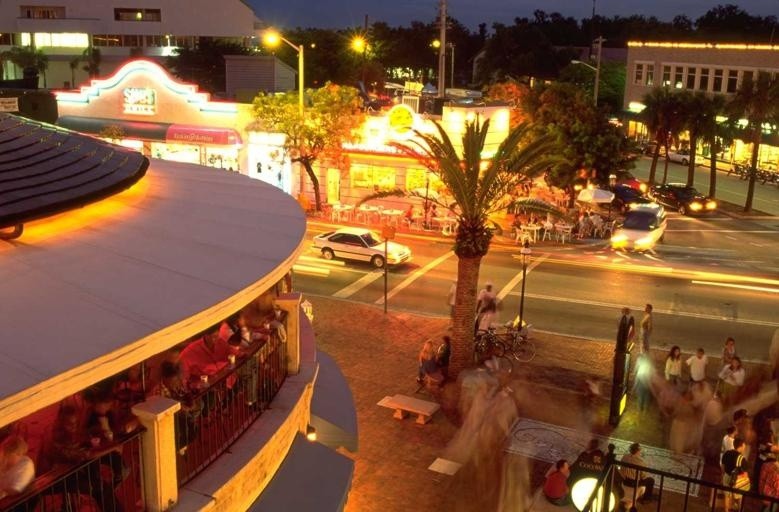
313 226 412 270
668 148 706 167
592 177 667 254
647 141 665 155
646 181 718 217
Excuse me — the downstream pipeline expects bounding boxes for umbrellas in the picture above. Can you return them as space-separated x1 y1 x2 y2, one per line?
577 188 615 217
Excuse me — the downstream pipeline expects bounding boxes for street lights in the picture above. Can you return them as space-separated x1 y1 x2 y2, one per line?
570 59 599 109
379 224 399 313
433 41 454 89
515 239 530 330
263 28 311 210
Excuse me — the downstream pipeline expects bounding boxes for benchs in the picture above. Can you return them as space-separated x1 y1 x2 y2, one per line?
376 393 440 427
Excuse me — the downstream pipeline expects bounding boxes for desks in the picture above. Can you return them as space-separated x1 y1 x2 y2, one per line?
334 204 456 230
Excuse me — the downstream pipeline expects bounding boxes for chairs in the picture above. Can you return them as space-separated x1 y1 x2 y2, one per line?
515 220 618 246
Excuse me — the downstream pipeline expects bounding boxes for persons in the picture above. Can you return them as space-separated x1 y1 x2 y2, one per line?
404 203 604 245
0 309 288 512
416 281 779 511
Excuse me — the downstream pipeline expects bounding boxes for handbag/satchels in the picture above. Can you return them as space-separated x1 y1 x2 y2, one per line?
732 472 751 500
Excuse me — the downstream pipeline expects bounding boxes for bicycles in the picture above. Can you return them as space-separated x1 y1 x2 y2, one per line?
472 326 538 376
727 162 779 185
